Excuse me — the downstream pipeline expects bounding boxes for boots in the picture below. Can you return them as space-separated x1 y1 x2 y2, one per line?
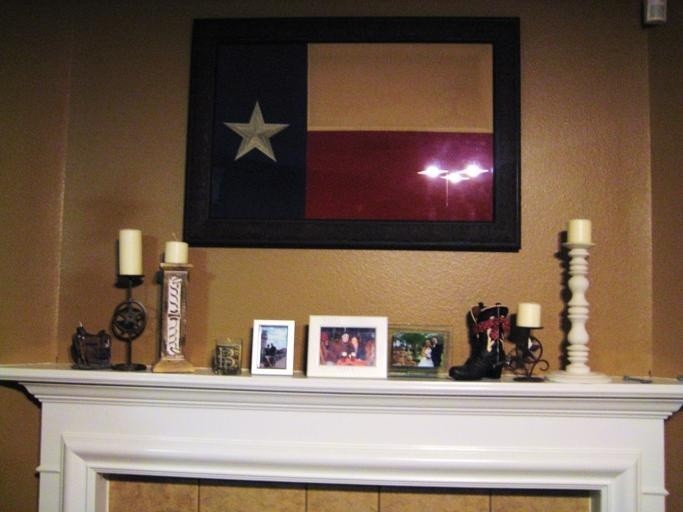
449 302 509 381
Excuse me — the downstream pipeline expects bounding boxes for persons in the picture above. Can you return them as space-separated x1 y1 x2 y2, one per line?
263 344 271 364
431 335 443 367
335 332 355 360
419 339 434 368
268 342 277 368
349 335 365 358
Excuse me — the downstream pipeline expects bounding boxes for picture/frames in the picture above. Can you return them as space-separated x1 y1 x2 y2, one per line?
181 17 522 254
249 314 455 381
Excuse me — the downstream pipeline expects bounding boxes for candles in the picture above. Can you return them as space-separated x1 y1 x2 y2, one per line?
567 216 592 248
117 228 189 276
515 303 542 328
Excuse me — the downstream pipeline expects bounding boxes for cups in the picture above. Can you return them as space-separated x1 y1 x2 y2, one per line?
212 336 244 376
